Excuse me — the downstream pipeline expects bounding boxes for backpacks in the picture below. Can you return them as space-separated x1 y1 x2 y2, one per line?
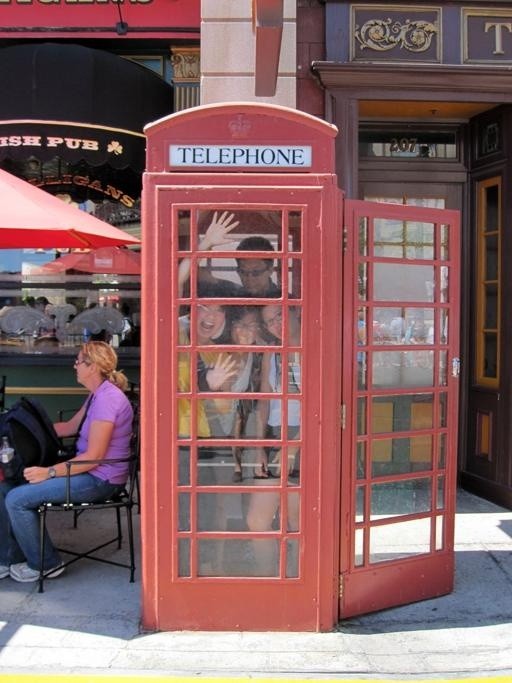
0 393 64 488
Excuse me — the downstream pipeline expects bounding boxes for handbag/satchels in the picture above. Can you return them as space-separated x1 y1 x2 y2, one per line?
231 412 302 486
210 436 240 486
61 443 77 463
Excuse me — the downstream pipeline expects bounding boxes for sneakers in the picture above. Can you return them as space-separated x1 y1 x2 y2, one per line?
9 560 66 584
0 565 10 580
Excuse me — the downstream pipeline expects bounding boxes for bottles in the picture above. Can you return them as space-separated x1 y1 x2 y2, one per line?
0 435 14 463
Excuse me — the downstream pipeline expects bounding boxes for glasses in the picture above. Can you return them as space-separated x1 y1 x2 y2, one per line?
235 265 269 278
75 359 86 367
232 318 262 332
261 312 282 329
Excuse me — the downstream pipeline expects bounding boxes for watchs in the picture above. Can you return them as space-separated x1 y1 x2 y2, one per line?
48 467 56 478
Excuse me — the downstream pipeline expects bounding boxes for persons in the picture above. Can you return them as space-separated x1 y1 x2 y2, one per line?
4 341 135 583
0 296 140 347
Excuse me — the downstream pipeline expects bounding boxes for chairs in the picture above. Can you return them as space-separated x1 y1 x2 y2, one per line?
31 412 142 593
